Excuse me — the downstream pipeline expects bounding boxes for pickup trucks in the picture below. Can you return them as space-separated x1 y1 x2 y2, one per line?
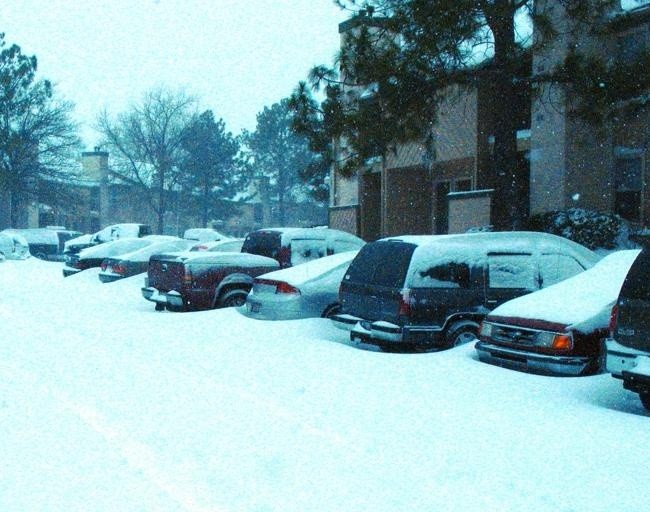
142 228 369 313
63 222 153 259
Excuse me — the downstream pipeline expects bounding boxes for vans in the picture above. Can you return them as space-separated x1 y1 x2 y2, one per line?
336 230 605 354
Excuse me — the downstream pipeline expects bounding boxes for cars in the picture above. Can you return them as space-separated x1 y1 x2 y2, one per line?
182 227 233 241
97 239 207 282
243 250 360 321
186 238 247 253
0 225 80 260
60 237 158 278
472 240 649 414
